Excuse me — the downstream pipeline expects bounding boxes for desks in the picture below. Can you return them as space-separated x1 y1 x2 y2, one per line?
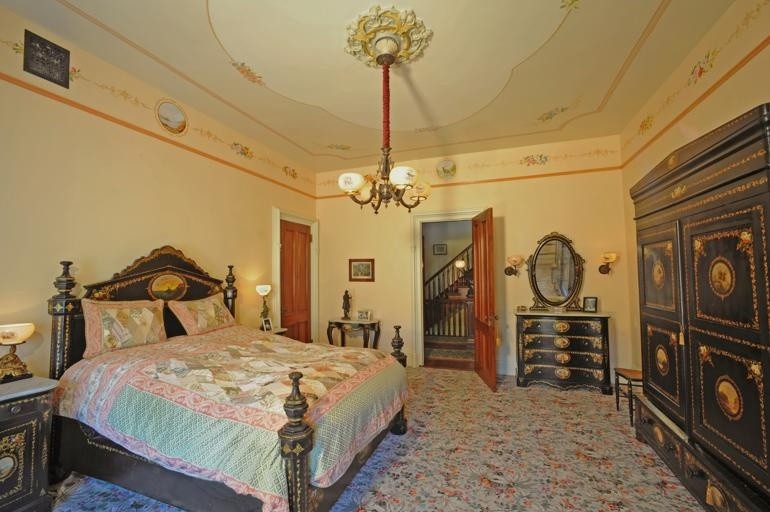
513 311 614 396
326 319 381 350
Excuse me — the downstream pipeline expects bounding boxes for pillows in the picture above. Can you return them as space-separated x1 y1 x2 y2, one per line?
78 295 168 359
167 291 244 337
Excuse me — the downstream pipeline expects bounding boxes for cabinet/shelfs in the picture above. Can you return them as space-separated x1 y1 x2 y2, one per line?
627 101 770 512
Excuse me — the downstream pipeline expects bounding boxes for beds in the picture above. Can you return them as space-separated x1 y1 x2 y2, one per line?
45 244 411 511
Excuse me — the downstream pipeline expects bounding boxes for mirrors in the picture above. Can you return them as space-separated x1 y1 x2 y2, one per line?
524 230 586 311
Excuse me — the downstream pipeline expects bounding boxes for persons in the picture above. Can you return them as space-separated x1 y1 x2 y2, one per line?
358 311 368 319
341 290 353 320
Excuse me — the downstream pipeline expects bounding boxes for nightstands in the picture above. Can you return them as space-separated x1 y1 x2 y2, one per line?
273 327 289 337
0 376 61 511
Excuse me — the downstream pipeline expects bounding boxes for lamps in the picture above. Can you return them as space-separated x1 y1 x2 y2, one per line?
255 280 273 331
0 322 36 385
337 3 434 215
454 257 474 298
597 251 618 275
503 255 523 277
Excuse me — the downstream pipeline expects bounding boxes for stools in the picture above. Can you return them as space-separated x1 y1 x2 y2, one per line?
613 366 644 427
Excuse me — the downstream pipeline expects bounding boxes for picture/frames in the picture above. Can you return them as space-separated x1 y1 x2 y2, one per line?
583 296 599 313
22 26 70 89
432 243 448 256
348 258 375 283
